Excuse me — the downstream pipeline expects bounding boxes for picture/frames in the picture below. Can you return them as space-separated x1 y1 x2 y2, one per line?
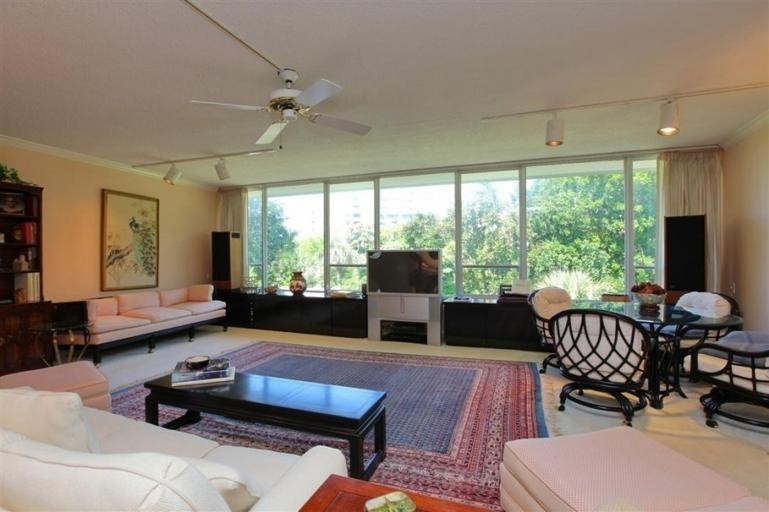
101 189 159 291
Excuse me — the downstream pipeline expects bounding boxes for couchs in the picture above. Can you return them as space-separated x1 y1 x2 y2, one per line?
498 424 768 512
1 360 110 413
0 389 345 510
53 285 227 365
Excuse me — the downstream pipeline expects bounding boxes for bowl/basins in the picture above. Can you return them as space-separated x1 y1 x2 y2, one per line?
266 287 278 293
185 356 209 369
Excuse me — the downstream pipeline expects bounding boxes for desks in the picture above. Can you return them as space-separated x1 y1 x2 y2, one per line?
298 474 491 511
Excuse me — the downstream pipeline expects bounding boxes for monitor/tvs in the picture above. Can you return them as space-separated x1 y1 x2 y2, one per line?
366 248 442 296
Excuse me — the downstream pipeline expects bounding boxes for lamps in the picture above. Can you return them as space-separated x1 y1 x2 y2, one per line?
545 111 564 147
163 164 181 185
214 158 231 181
657 98 680 137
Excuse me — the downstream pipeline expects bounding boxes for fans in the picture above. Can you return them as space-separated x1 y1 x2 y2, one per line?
188 69 371 146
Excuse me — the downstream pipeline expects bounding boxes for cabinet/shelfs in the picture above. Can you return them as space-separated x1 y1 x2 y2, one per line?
213 290 367 339
0 182 52 374
367 292 443 346
442 298 554 352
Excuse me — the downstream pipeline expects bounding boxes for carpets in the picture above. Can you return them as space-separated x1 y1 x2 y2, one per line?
110 342 548 510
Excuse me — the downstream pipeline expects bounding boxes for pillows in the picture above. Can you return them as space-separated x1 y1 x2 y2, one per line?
0 391 94 451
194 462 258 511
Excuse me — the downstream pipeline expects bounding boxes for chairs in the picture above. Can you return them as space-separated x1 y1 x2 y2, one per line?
602 294 632 302
690 329 769 427
528 287 570 374
674 291 739 382
548 309 650 427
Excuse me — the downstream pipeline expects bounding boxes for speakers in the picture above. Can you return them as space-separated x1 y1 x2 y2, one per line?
211 231 242 290
664 214 709 305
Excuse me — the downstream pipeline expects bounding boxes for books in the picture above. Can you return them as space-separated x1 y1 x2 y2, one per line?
23 222 38 246
171 355 236 389
15 271 41 305
